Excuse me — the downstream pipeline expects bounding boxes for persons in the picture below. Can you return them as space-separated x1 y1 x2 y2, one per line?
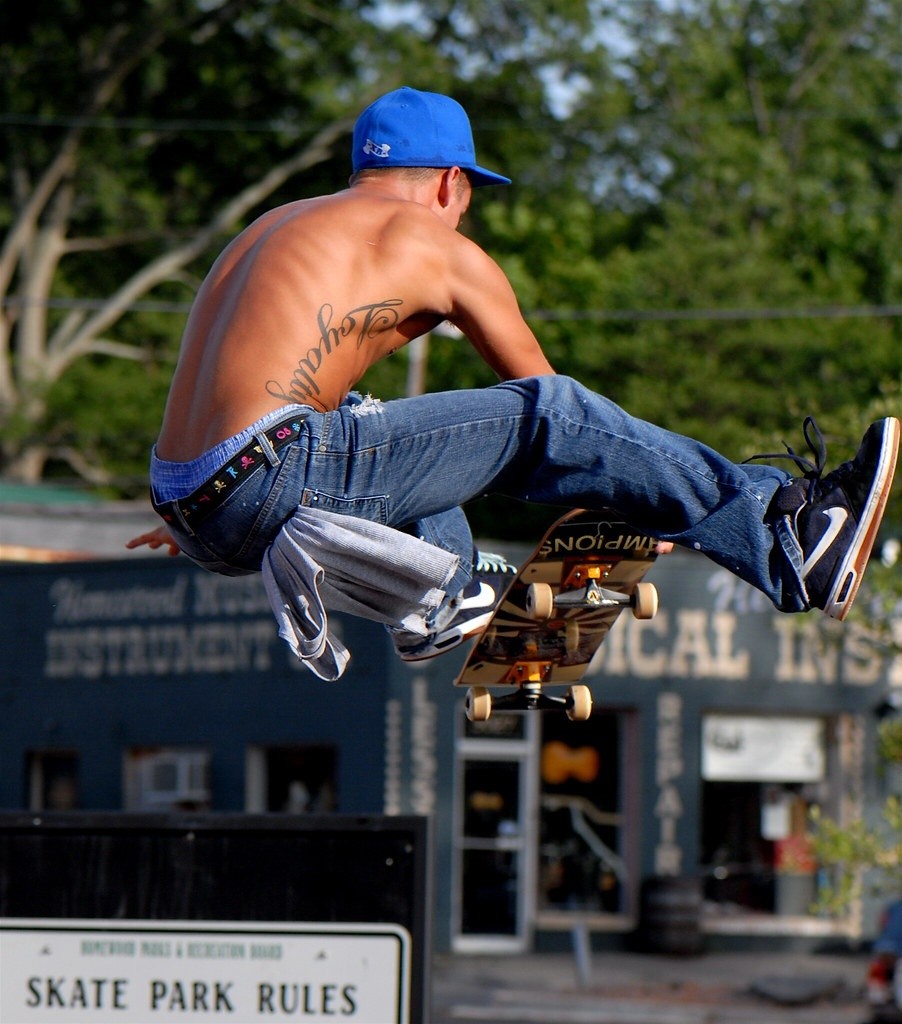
126 88 902 686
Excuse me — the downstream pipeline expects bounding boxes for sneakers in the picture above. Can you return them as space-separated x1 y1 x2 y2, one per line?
740 416 901 622
391 550 520 663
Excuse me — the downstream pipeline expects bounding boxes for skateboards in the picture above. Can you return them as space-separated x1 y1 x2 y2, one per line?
453 507 660 724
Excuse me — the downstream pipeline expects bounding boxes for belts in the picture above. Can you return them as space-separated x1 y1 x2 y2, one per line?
150 414 308 532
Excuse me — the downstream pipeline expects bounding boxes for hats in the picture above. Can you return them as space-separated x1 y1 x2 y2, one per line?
351 85 513 188
